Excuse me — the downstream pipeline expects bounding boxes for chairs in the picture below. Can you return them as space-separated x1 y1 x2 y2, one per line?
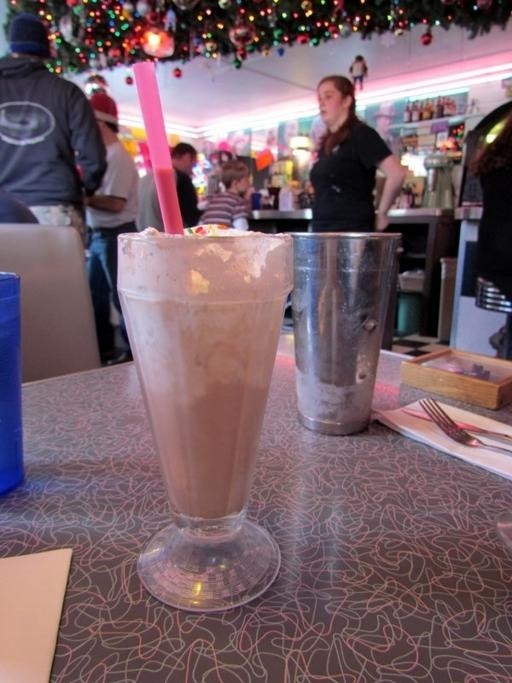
0 220 101 386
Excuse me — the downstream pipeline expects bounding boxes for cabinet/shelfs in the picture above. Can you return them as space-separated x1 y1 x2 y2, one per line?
248 213 460 338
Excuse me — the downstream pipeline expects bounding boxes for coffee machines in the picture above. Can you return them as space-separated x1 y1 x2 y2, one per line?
421 152 455 210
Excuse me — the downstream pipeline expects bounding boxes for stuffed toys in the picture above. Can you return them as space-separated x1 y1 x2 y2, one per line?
349 55 368 90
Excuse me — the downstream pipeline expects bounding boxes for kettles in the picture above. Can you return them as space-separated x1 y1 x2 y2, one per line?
396 187 416 208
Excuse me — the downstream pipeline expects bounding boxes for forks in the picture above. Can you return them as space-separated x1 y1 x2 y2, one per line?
419 393 511 457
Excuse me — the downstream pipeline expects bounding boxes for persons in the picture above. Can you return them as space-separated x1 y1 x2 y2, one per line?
372 104 400 163
471 117 511 359
87 91 136 365
137 141 252 233
1 14 108 250
304 76 406 325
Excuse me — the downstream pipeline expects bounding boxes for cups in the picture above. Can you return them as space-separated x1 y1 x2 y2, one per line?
112 231 295 610
252 192 262 209
292 229 399 434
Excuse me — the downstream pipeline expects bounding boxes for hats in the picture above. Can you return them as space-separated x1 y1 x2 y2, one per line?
89 94 118 125
9 14 49 55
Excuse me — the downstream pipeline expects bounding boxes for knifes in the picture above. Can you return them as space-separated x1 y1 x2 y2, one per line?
403 407 511 445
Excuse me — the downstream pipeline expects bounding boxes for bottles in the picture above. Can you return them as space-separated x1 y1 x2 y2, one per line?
402 94 459 121
0 268 27 499
